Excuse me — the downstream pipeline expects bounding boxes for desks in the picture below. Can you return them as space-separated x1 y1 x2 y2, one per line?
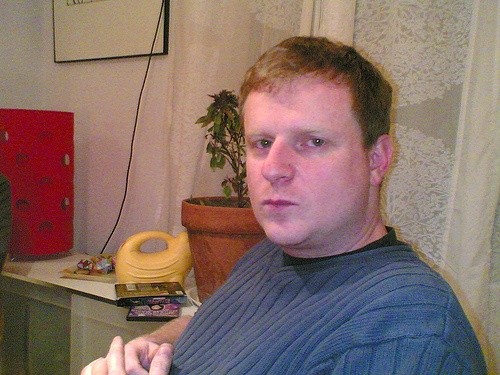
0 252 201 375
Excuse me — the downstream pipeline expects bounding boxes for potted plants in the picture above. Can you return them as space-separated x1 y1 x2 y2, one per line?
182 89 266 303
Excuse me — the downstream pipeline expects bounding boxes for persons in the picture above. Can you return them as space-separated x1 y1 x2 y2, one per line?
81 35 489 375
